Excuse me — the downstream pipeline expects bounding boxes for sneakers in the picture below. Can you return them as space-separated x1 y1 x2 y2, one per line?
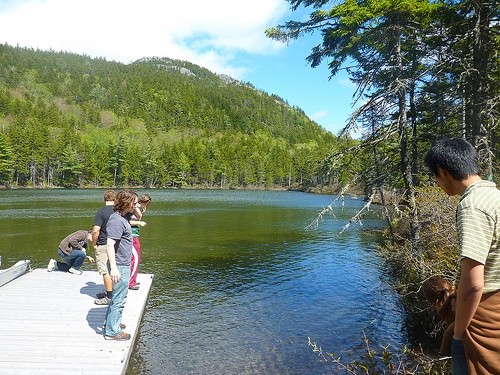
48 258 56 271
69 267 82 275
96 290 107 298
105 330 130 341
95 296 112 305
103 323 126 329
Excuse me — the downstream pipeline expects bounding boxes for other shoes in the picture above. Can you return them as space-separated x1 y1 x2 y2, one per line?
131 286 139 290
136 282 140 285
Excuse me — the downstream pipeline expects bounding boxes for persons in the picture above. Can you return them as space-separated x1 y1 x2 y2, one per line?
423 137 500 375
91 190 116 305
101 190 138 340
47 227 95 276
129 194 152 290
